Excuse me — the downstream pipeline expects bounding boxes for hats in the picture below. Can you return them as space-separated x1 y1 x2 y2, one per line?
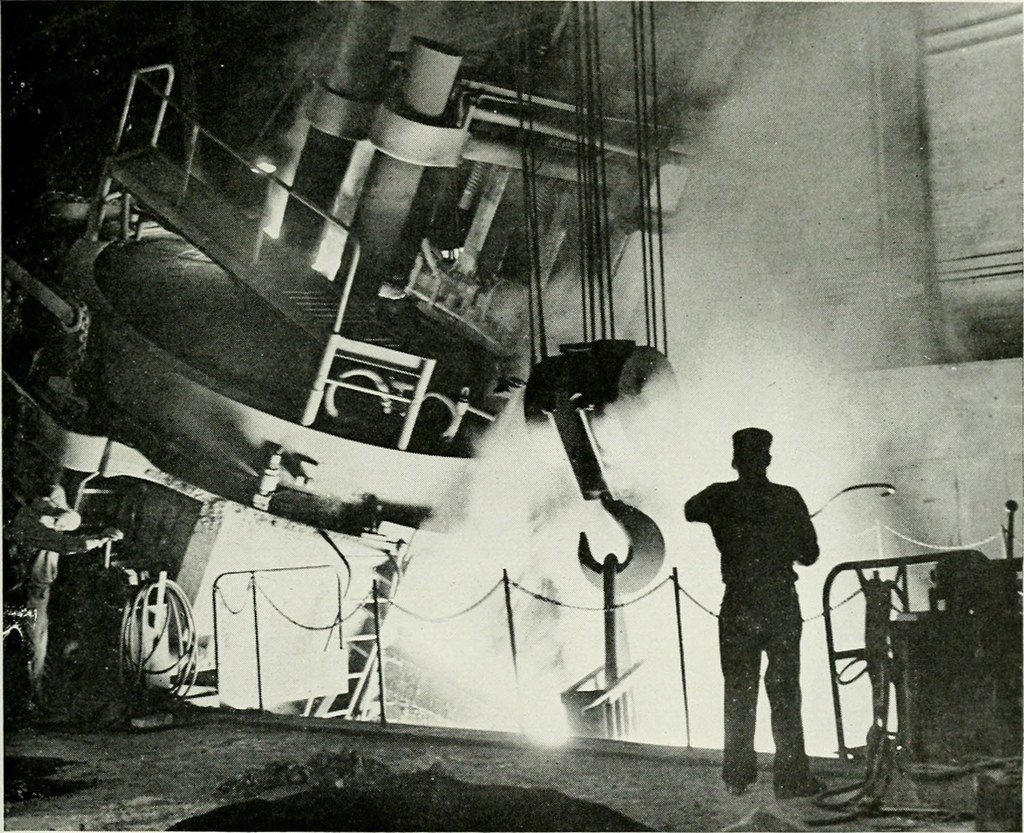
35 481 73 513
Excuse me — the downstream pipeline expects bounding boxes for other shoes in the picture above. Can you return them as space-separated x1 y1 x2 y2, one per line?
722 773 758 794
775 779 827 798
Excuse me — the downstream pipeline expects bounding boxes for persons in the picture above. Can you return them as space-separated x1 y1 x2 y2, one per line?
684 427 820 801
